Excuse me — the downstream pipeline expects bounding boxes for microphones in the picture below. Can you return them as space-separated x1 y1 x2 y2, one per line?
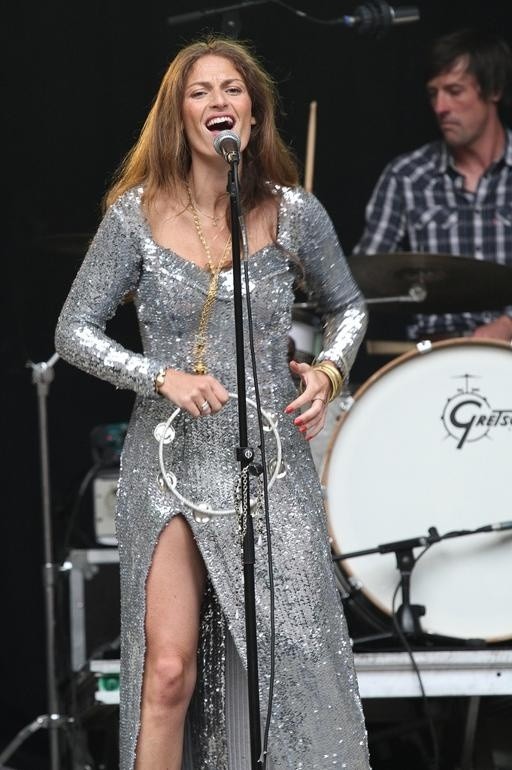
343 0 427 35
213 129 241 165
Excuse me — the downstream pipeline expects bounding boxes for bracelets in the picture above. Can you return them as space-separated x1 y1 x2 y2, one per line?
299 361 343 403
156 369 166 394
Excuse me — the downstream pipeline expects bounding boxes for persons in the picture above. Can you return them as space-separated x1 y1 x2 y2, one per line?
349 30 511 345
54 36 378 768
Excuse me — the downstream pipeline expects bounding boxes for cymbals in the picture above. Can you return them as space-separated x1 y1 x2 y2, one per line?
341 253 512 314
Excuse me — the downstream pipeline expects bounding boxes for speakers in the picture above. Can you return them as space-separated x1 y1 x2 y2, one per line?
67 548 121 769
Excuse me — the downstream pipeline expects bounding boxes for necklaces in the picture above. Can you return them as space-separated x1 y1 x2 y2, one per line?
195 206 229 226
183 177 231 375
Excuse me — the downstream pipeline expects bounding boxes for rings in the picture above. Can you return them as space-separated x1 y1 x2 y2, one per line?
199 400 210 411
312 396 327 405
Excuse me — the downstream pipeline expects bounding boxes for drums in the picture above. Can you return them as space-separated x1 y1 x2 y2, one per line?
299 385 358 483
288 321 328 382
318 334 512 644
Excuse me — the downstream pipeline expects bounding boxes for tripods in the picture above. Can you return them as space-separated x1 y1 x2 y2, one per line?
332 520 493 649
1 354 92 770
